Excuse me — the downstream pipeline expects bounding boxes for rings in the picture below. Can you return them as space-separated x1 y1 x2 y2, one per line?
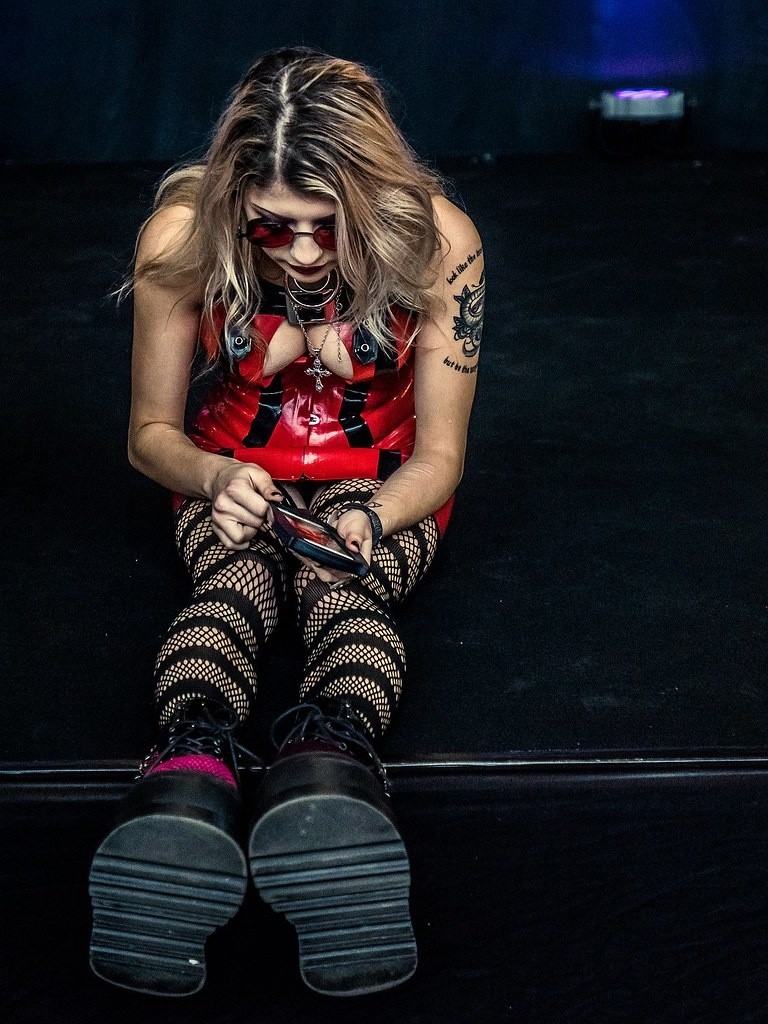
326 577 353 590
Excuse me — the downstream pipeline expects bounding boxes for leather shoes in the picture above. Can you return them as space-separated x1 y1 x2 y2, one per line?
252 695 420 995
85 704 248 995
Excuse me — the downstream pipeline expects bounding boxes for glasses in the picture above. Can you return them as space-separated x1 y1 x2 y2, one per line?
236 203 345 251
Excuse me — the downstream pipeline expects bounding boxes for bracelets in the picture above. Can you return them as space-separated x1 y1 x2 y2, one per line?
327 502 383 546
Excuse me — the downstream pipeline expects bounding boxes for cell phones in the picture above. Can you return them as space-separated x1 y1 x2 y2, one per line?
269 499 372 579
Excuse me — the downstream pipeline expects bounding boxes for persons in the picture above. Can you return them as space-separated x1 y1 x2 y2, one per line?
86 42 498 1000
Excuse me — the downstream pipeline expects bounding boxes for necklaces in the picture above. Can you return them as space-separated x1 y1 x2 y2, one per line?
276 266 348 392
